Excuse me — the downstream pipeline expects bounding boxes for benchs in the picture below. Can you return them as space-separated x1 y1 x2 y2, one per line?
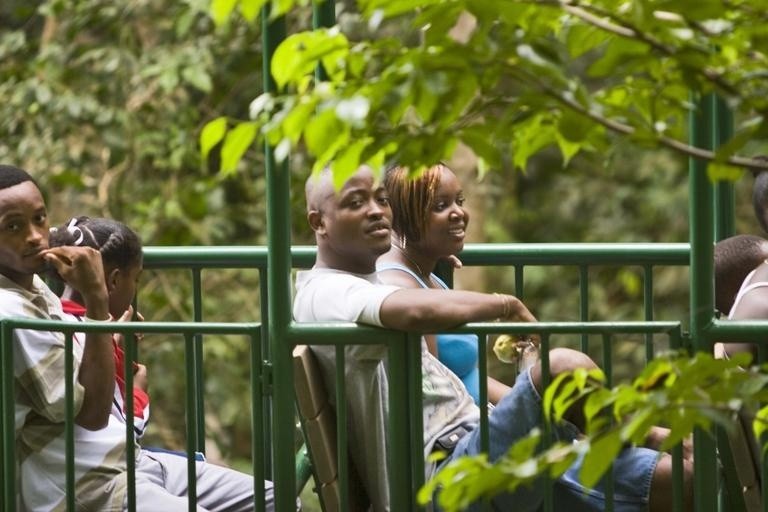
292 341 366 510
713 341 764 509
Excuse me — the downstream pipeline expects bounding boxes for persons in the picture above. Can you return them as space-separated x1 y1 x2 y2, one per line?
376 161 697 464
292 164 696 511
712 233 768 316
0 162 303 512
722 171 768 378
47 215 208 464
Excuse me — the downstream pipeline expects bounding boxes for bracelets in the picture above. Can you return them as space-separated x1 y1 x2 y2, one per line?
84 312 113 323
493 292 511 323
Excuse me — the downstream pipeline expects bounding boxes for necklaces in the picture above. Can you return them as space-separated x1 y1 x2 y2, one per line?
390 242 441 290
